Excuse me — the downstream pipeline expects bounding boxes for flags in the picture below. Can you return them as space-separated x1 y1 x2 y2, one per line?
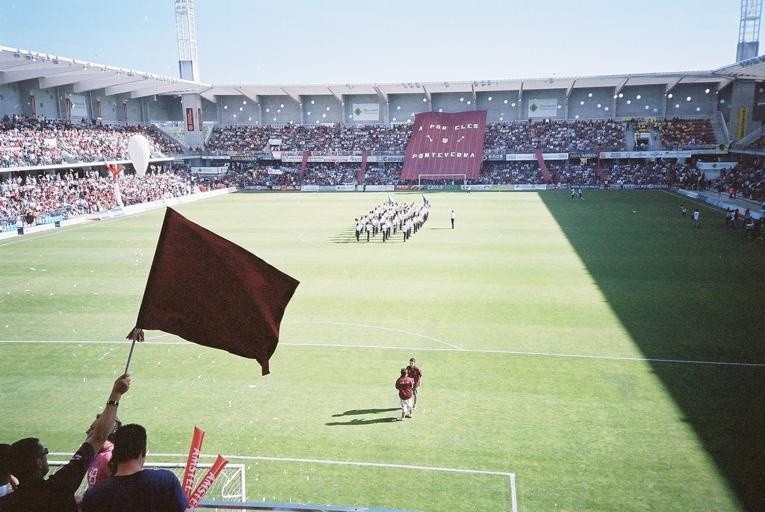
134 207 300 377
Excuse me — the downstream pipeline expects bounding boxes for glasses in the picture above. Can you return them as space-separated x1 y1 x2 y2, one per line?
41 448 48 454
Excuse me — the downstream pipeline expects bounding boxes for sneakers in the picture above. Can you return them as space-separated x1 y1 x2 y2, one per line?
397 408 416 421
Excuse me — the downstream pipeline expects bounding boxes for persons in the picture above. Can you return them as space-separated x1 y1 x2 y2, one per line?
74 423 195 511
570 188 577 200
577 187 585 201
680 137 765 241
405 357 423 409
74 412 123 512
395 367 415 421
1 113 192 231
0 442 19 495
356 196 433 241
193 118 712 193
0 374 132 512
449 209 456 229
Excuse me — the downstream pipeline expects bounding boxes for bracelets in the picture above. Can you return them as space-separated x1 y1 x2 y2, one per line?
106 399 119 405
